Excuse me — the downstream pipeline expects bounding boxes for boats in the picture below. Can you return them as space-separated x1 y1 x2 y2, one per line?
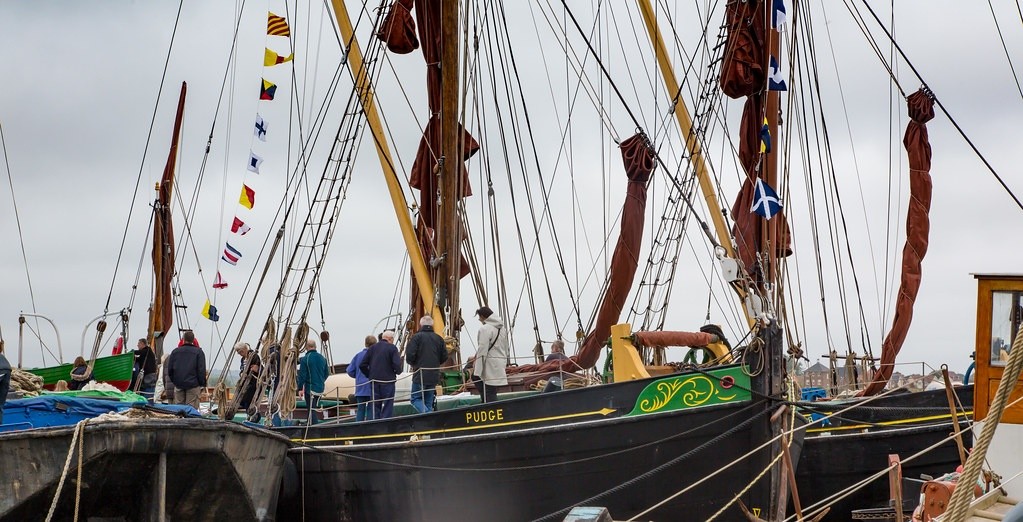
18 309 136 393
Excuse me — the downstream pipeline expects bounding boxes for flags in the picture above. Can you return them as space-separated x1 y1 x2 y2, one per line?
771 0 787 29
221 243 242 266
231 217 251 235
248 150 263 175
265 48 294 66
259 79 277 101
750 177 783 219
202 298 219 323
253 113 269 142
267 12 291 37
759 117 771 153
212 270 228 290
238 184 256 209
768 56 787 91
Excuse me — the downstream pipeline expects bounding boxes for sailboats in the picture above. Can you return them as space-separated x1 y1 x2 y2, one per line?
0 1 1023 522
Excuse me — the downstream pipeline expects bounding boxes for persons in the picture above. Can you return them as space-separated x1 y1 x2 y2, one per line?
359 329 403 420
406 316 449 414
235 340 329 425
0 340 13 426
133 332 207 410
547 342 568 361
471 307 510 404
53 380 69 392
69 356 95 389
346 335 377 421
